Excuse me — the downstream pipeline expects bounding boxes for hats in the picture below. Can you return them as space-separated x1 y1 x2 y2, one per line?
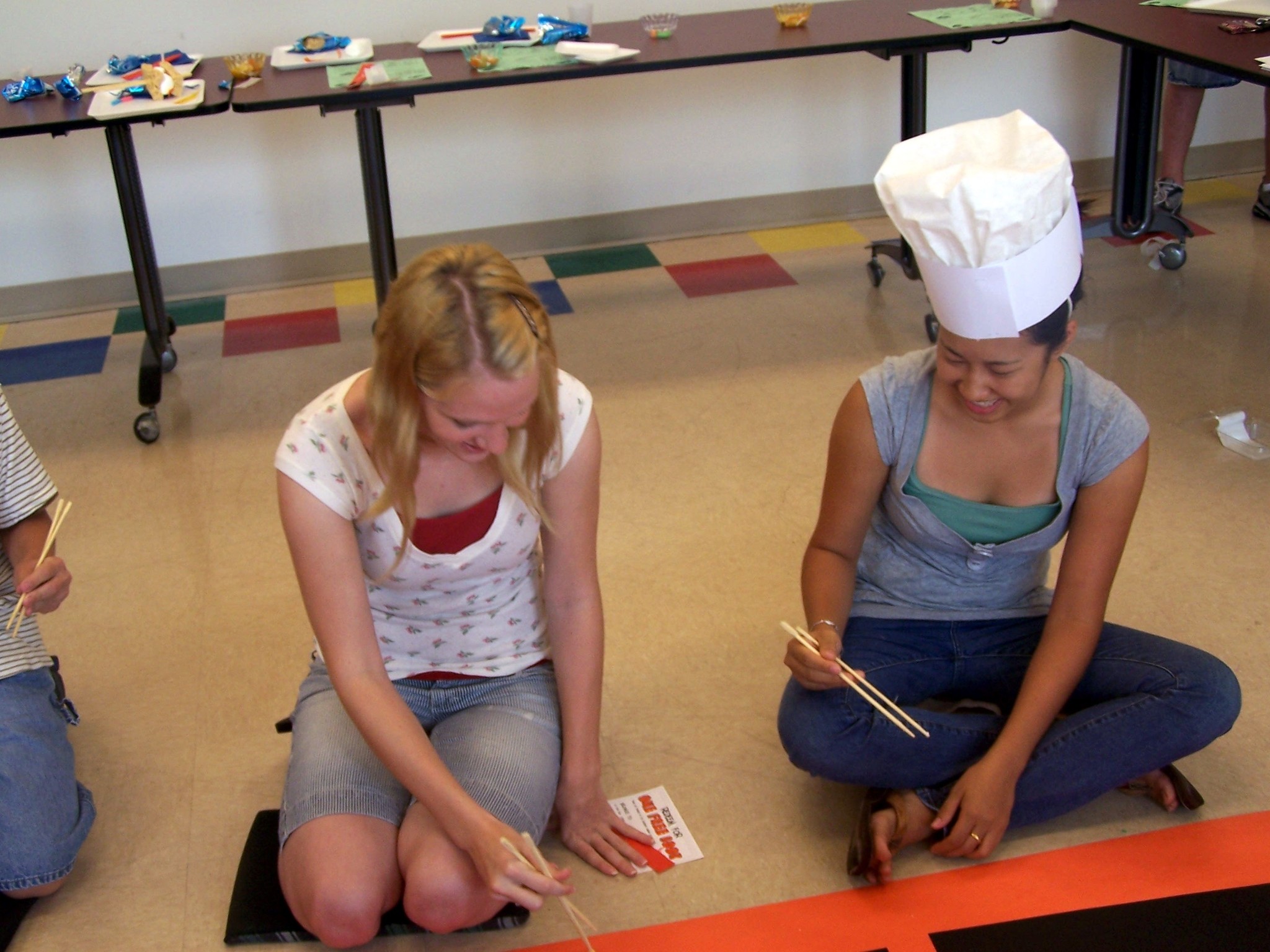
874 108 1083 341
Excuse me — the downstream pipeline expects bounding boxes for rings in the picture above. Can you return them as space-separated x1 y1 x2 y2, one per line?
971 830 986 844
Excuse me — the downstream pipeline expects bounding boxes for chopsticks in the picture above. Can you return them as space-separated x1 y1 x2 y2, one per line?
6 498 73 639
499 831 598 952
779 620 930 738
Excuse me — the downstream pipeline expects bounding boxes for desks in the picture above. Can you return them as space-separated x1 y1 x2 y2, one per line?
0 0 1270 448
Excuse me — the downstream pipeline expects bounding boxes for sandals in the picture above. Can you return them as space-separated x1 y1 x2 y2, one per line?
1116 761 1205 813
847 784 901 881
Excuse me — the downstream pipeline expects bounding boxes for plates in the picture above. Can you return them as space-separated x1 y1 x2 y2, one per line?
270 38 374 71
417 26 540 53
87 79 204 120
85 54 202 85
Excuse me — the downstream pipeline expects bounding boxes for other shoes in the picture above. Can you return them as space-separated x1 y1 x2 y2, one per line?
1151 177 1184 215
1252 183 1270 222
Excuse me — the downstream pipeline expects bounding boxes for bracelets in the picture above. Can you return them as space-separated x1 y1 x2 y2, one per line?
809 617 845 636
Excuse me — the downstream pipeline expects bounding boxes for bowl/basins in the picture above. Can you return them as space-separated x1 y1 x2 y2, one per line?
991 0 1020 9
223 52 267 79
461 42 504 72
639 13 680 39
774 2 813 28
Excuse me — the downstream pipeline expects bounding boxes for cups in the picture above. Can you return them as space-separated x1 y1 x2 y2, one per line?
568 3 593 38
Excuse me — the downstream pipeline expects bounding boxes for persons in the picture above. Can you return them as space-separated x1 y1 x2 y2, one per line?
0 383 96 900
776 108 1243 890
275 242 656 949
